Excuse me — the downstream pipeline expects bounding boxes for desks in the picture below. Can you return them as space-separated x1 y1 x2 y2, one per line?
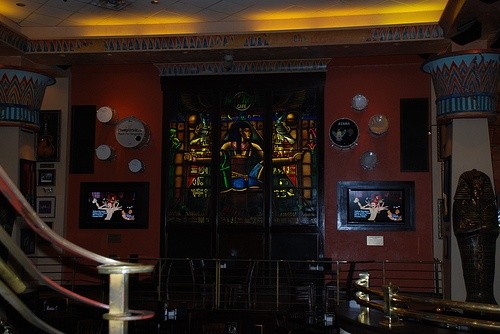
202 264 246 310
296 266 340 313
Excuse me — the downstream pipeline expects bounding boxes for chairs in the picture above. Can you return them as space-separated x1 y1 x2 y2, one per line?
134 256 170 304
324 261 355 310
228 260 259 309
101 255 122 299
279 257 315 310
187 256 216 309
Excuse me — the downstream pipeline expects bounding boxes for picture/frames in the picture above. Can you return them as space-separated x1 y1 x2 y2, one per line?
36 109 62 163
336 181 417 233
37 195 58 219
20 228 36 255
39 221 55 247
79 181 149 229
38 167 58 187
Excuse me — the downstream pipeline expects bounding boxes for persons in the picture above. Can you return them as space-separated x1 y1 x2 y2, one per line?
452 170 499 305
86 190 139 221
183 120 303 218
351 192 403 221
